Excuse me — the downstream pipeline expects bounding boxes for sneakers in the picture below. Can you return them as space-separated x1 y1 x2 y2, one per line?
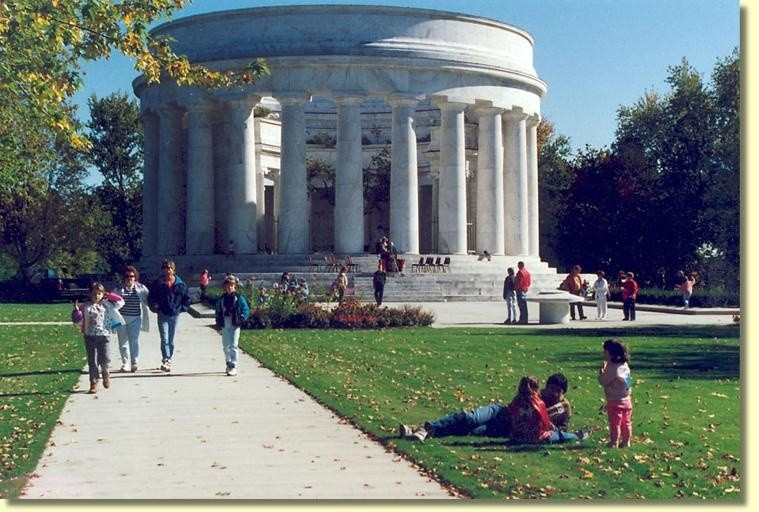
578 425 592 443
400 423 414 439
132 365 138 373
412 427 429 443
225 363 238 376
161 361 172 372
121 364 129 373
103 374 111 388
89 382 98 393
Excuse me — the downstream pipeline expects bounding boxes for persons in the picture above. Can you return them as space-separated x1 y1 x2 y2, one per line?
111 266 159 372
564 264 696 321
199 225 397 306
72 283 127 394
401 374 571 442
147 258 191 372
509 376 593 445
215 281 250 376
514 262 530 324
503 268 516 324
599 339 633 448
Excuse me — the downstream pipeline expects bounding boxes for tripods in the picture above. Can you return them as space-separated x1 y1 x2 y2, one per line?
384 257 403 286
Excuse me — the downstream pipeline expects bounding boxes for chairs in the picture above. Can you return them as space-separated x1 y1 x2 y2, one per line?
307 255 451 273
